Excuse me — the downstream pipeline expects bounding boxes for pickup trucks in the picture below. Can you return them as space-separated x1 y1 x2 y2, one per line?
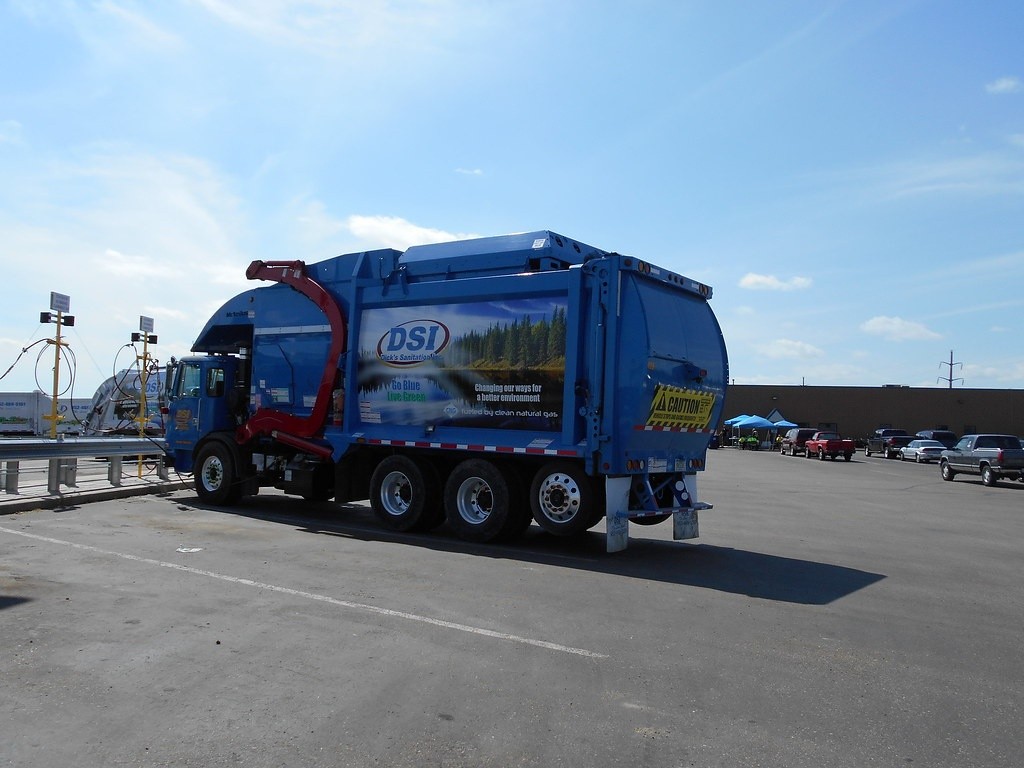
938 433 1024 489
804 427 855 461
865 427 914 459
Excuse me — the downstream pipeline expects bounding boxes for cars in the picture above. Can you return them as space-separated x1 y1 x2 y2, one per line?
902 440 949 462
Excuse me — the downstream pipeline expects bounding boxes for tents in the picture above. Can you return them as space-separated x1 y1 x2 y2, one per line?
724 415 798 451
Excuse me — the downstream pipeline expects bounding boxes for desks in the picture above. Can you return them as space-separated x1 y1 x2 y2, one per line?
729 438 740 448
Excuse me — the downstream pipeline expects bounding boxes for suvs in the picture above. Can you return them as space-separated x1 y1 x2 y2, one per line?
776 426 817 454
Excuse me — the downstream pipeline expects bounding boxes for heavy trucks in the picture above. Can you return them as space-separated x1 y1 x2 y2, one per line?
154 225 736 551
